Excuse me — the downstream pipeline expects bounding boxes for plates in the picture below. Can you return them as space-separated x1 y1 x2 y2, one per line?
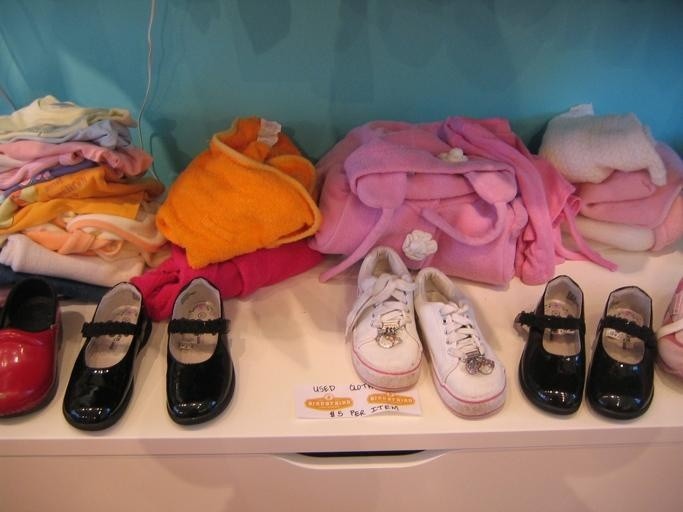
0 245 683 512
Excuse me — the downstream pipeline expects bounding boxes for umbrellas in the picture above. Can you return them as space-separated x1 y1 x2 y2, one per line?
519 276 587 413
586 288 657 418
165 276 237 421
2 276 63 417
61 281 152 430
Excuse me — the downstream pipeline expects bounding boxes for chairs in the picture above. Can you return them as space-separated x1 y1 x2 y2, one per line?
415 269 509 419
659 275 683 378
354 245 423 392
